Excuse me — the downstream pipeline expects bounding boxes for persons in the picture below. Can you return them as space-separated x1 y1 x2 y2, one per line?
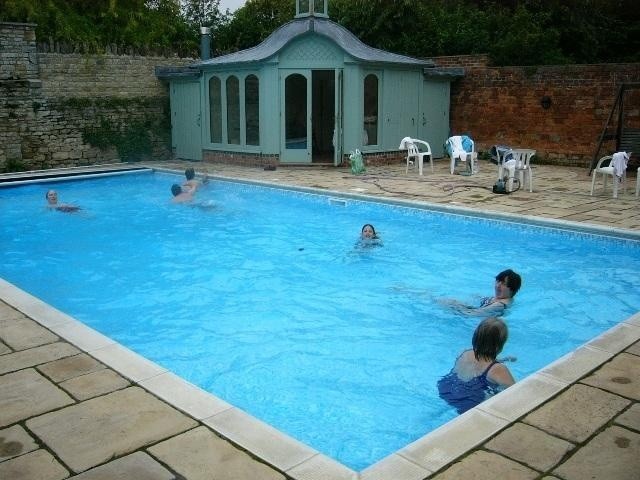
438 270 521 320
46 190 79 212
182 168 208 187
171 184 195 202
362 224 375 240
437 317 517 414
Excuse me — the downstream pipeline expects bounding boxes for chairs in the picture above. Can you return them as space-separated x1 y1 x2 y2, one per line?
446 136 474 174
636 167 640 197
406 139 433 175
502 149 536 192
591 152 631 198
495 144 523 182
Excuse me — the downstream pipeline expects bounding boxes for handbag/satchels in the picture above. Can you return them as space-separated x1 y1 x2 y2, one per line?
349 148 366 174
492 176 520 194
